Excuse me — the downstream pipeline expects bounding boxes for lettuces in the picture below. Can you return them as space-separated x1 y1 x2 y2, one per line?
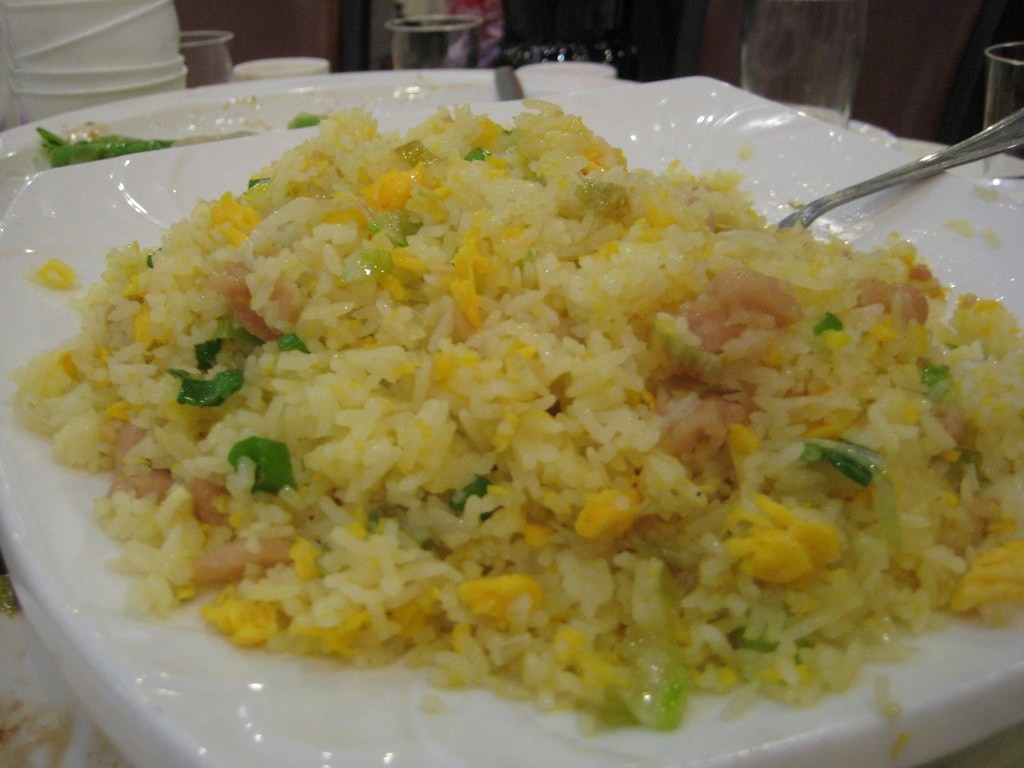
35 126 177 168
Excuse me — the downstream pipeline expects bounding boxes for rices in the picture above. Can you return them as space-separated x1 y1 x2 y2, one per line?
1 96 1024 740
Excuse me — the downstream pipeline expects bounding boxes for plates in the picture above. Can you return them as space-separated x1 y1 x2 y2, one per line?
1 70 638 226
0 75 1023 768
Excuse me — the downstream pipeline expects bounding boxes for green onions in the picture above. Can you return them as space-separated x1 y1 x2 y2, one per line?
169 112 952 495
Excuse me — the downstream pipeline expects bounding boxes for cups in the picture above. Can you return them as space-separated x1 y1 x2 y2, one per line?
982 40 1024 199
0 0 622 132
